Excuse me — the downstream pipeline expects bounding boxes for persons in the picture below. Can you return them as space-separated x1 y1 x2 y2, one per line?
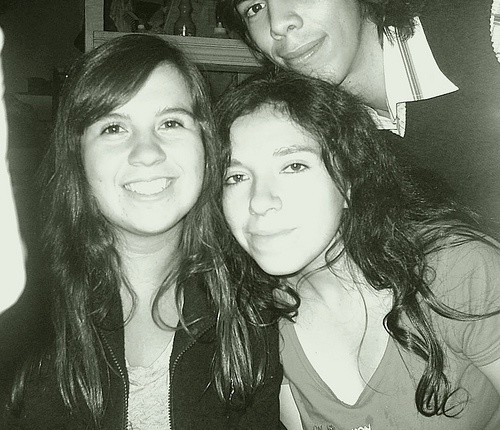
215 0 500 244
1 35 289 429
210 69 500 430
